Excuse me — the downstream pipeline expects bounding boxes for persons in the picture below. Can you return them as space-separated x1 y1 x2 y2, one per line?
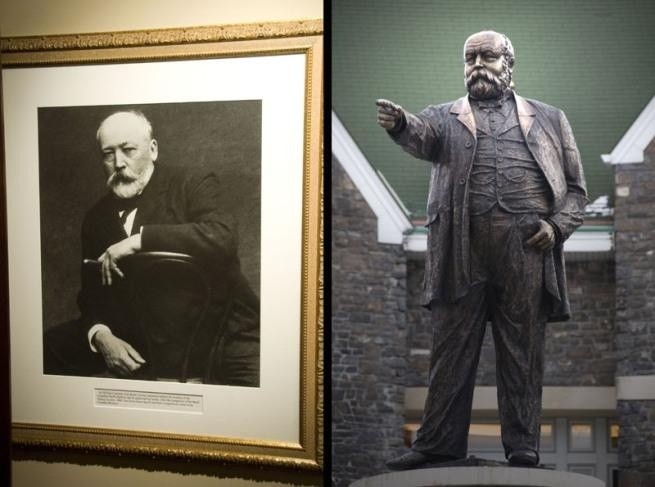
42 98 259 390
374 30 590 469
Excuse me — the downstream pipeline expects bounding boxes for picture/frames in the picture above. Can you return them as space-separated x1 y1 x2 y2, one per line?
1 21 329 477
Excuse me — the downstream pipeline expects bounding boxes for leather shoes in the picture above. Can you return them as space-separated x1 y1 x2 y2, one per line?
506 448 538 467
384 450 467 471
